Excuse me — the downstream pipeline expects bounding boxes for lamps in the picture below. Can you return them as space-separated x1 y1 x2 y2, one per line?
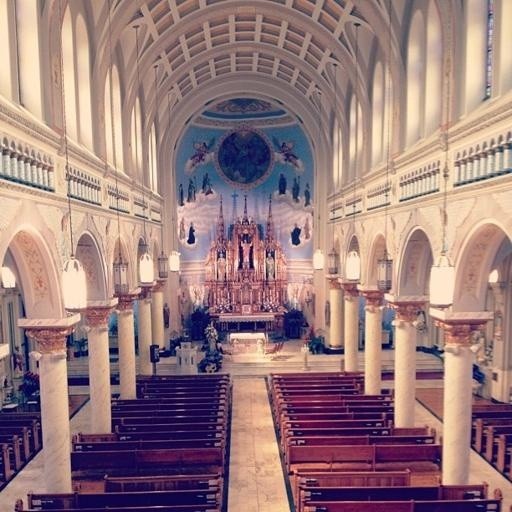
56 2 180 314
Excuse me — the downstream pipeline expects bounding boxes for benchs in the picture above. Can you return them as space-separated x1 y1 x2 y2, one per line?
1 367 234 512
268 371 512 510
